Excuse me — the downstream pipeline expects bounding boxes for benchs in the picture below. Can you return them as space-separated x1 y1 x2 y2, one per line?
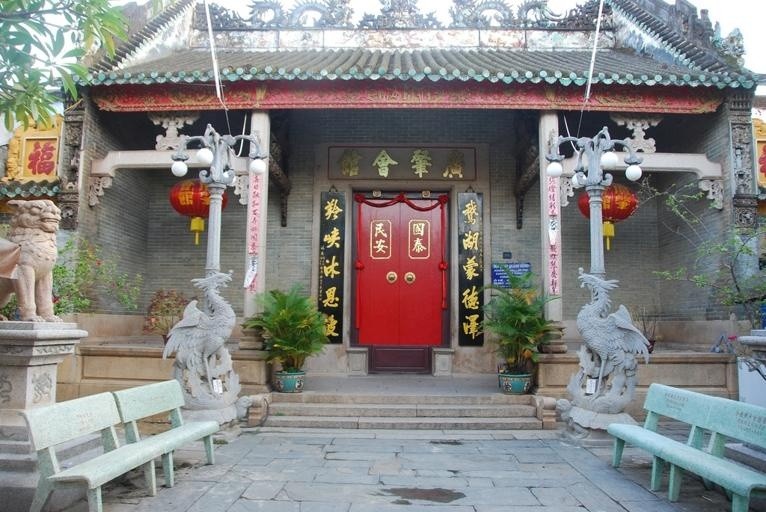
607 382 765 510
16 377 221 511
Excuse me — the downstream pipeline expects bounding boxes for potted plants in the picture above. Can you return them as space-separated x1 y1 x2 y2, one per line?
475 260 567 396
242 278 330 393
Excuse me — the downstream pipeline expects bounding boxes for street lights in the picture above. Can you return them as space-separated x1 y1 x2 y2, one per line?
544 127 639 317
170 123 269 317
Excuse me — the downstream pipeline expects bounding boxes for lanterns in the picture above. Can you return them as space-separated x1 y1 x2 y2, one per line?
579 173 639 252
170 169 228 246
